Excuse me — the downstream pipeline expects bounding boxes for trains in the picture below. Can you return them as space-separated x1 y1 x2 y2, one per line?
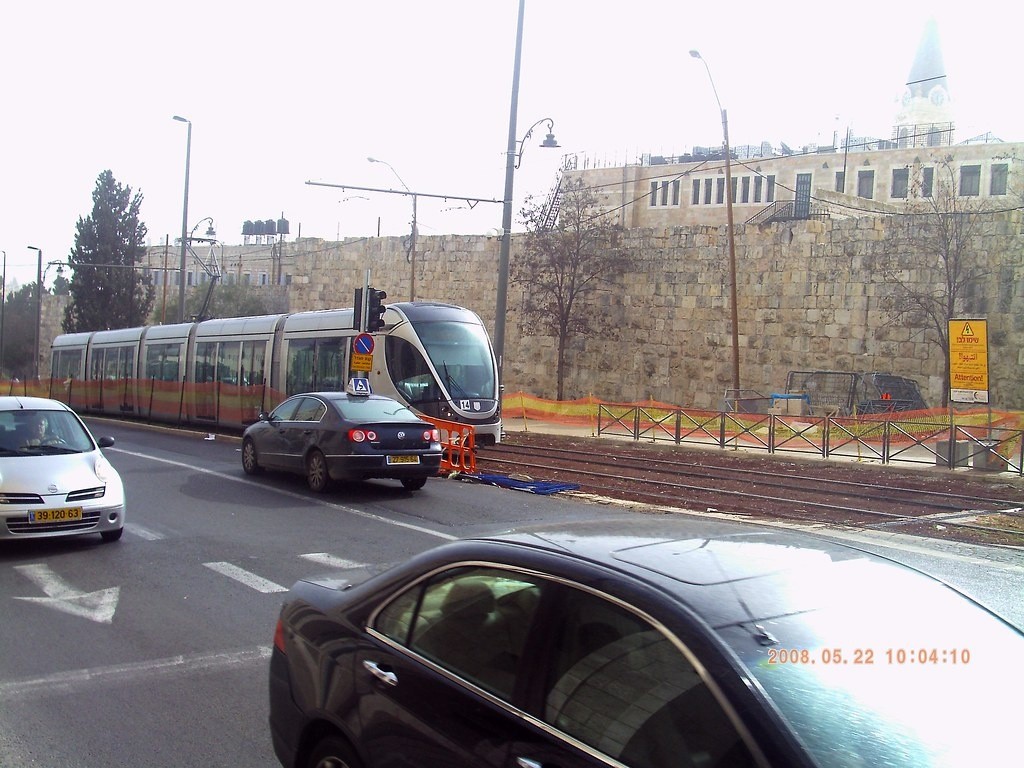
48 303 504 450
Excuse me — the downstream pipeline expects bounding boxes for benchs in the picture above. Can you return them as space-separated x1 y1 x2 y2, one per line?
398 582 644 702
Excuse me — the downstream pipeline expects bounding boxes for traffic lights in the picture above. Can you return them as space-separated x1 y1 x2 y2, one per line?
351 288 387 332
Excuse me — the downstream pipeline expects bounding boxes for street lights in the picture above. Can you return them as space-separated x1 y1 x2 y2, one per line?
494 1 562 413
366 157 418 303
171 114 218 323
27 244 64 385
688 47 742 416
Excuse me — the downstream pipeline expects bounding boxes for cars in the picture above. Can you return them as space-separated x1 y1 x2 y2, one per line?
240 388 445 493
269 516 1024 767
1 395 128 549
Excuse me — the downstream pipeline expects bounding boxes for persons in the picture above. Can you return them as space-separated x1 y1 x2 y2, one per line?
19 415 62 445
